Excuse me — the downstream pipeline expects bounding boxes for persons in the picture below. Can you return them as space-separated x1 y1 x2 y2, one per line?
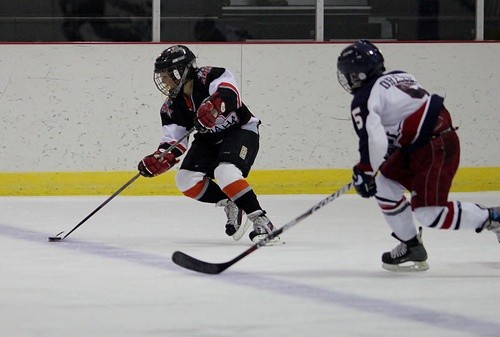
138 45 278 242
337 39 500 265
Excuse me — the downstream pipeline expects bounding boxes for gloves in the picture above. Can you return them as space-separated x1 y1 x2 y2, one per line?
193 91 225 132
137 144 181 178
352 163 377 199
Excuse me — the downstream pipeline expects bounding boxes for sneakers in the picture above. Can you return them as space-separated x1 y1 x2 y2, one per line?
475 203 500 244
247 210 280 246
381 226 429 272
215 198 252 241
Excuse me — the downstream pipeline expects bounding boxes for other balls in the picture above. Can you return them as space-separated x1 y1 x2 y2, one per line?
48 237 62 242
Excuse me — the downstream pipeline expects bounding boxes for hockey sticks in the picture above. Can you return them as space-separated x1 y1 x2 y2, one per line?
55 127 196 241
171 182 354 275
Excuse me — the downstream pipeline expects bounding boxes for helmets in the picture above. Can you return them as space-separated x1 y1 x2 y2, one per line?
337 39 386 94
153 45 197 102
